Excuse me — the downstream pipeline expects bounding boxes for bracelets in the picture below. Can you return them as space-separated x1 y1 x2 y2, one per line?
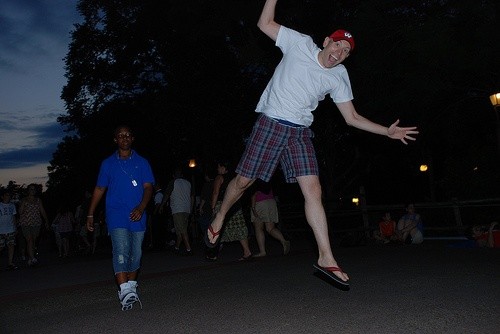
87 215 93 218
211 207 215 210
251 206 255 211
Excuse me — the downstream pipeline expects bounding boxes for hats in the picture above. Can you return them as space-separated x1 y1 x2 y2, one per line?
330 29 354 50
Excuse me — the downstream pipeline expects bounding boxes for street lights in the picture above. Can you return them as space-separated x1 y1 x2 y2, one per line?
186 158 201 239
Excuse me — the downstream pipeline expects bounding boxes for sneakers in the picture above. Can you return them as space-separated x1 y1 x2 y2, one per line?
169 248 193 256
8 264 18 270
117 280 142 312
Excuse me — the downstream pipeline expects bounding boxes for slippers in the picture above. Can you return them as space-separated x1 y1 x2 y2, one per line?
312 262 351 286
237 254 253 264
204 215 224 248
205 255 217 263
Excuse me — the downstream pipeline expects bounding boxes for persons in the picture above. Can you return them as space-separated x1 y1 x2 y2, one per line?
199 171 215 232
0 190 20 269
143 176 176 252
19 184 50 266
464 222 500 249
250 179 290 258
210 160 252 261
204 0 419 288
159 169 194 256
48 190 108 260
87 126 155 312
375 201 423 244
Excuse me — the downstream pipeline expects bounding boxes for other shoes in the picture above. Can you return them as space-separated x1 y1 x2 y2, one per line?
253 252 266 258
284 240 291 255
